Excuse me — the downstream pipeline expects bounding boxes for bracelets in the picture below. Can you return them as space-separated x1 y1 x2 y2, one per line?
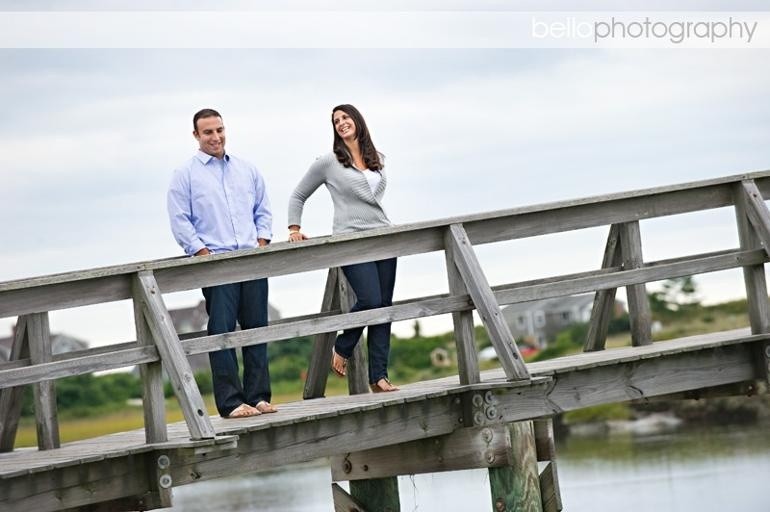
288 230 299 235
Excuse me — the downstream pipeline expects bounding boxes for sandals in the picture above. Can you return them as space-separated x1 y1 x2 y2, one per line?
329 342 347 379
256 399 278 414
224 403 262 419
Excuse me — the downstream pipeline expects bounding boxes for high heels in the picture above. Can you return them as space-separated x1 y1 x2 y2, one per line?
369 375 400 394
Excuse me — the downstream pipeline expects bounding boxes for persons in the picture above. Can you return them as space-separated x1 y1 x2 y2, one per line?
164 108 279 421
286 101 403 393
